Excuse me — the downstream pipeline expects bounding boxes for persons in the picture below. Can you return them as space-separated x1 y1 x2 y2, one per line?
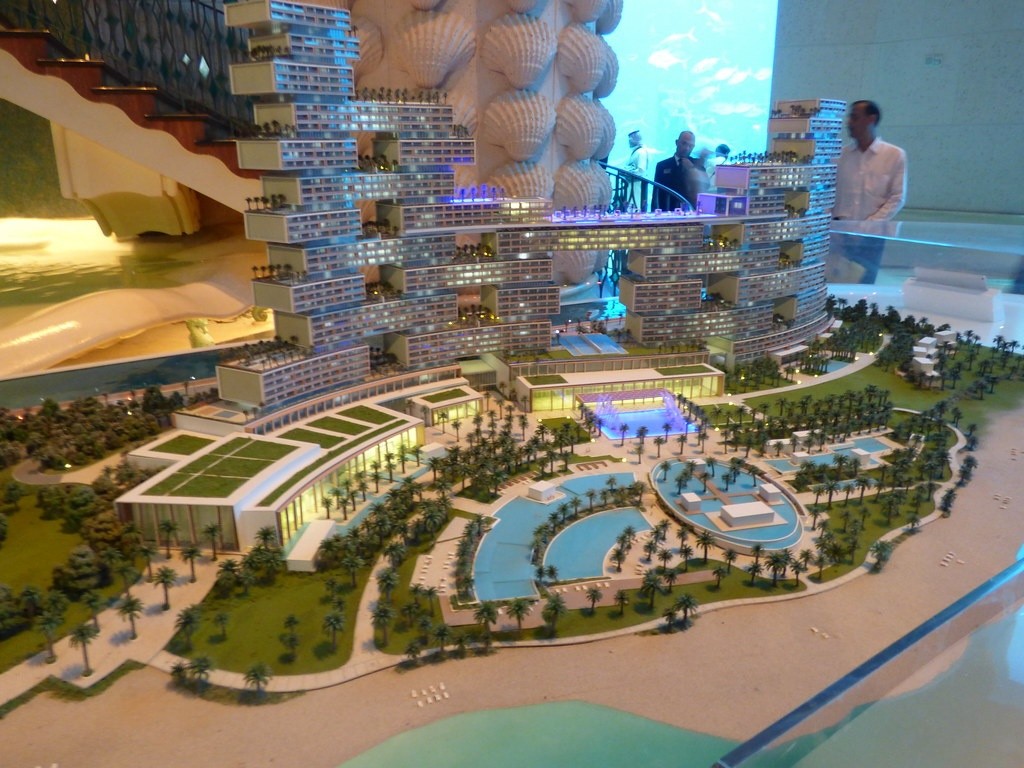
829 100 907 282
627 129 709 211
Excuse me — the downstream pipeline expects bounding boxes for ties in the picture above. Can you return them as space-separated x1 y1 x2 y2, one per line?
678 158 682 171
